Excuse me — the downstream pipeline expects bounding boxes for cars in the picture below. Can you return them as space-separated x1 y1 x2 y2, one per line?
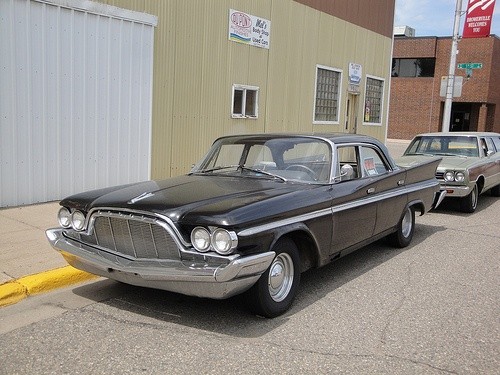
44 132 444 319
397 131 500 212
